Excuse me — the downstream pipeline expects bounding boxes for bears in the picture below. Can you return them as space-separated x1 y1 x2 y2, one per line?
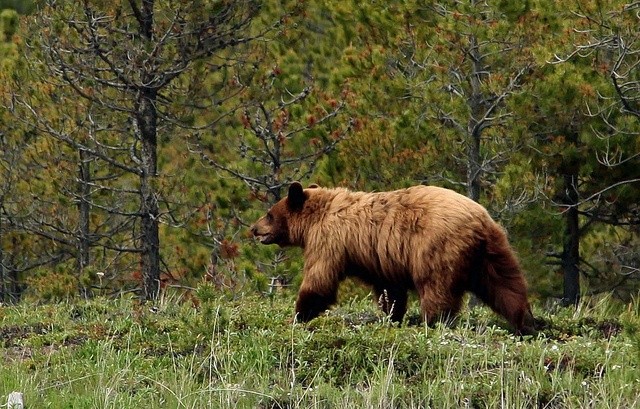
250 181 535 337
